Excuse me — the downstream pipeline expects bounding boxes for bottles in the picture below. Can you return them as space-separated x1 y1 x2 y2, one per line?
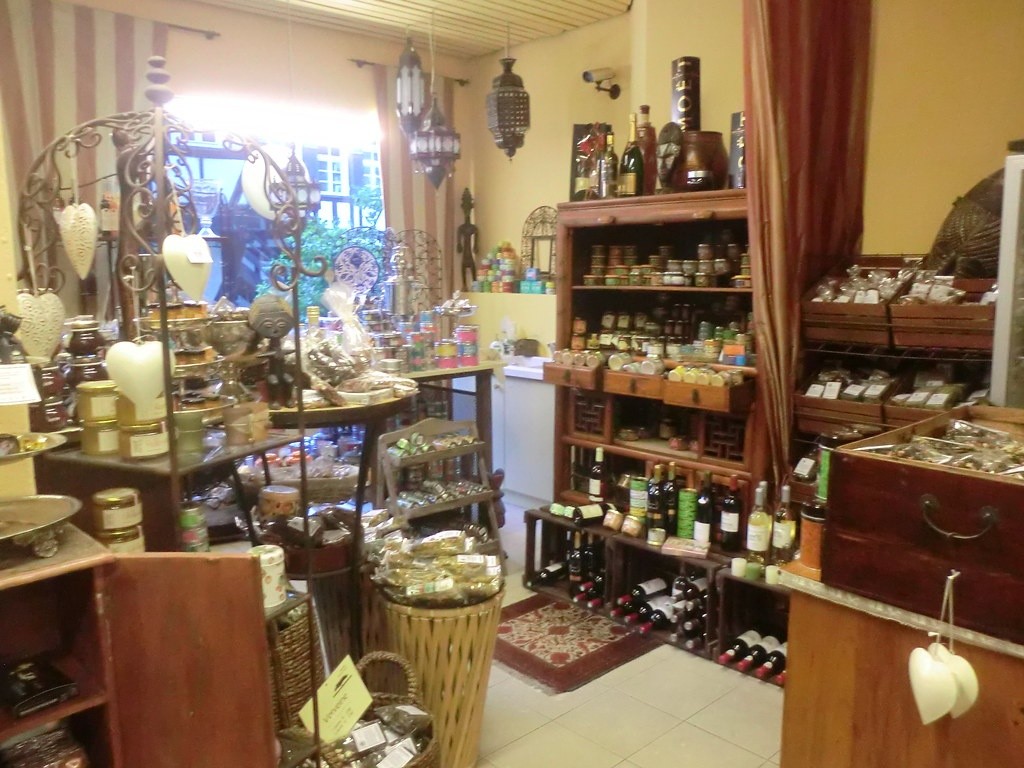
609 562 706 649
589 447 608 503
648 461 677 535
772 484 797 565
721 473 742 553
718 619 787 687
695 470 716 549
744 482 773 574
29 317 107 433
572 504 628 526
793 446 818 483
639 105 657 195
598 131 618 199
565 528 603 608
524 560 568 587
619 113 642 197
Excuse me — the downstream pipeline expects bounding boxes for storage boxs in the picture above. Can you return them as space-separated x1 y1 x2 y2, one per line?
818 404 1023 644
888 272 995 347
884 369 950 437
800 254 924 347
792 364 898 435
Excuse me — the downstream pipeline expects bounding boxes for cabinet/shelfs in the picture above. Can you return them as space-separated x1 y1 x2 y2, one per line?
0 325 506 768
786 349 995 507
525 188 790 690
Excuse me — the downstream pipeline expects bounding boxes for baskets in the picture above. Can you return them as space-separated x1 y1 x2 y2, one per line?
278 650 440 768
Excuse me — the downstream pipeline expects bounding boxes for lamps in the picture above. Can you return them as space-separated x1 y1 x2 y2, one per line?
485 28 530 163
408 15 461 188
396 35 426 139
268 143 321 234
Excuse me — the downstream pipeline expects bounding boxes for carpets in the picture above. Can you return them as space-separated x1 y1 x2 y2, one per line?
492 590 668 694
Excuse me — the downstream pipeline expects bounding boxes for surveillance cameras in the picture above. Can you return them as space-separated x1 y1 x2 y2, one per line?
583 68 614 83
